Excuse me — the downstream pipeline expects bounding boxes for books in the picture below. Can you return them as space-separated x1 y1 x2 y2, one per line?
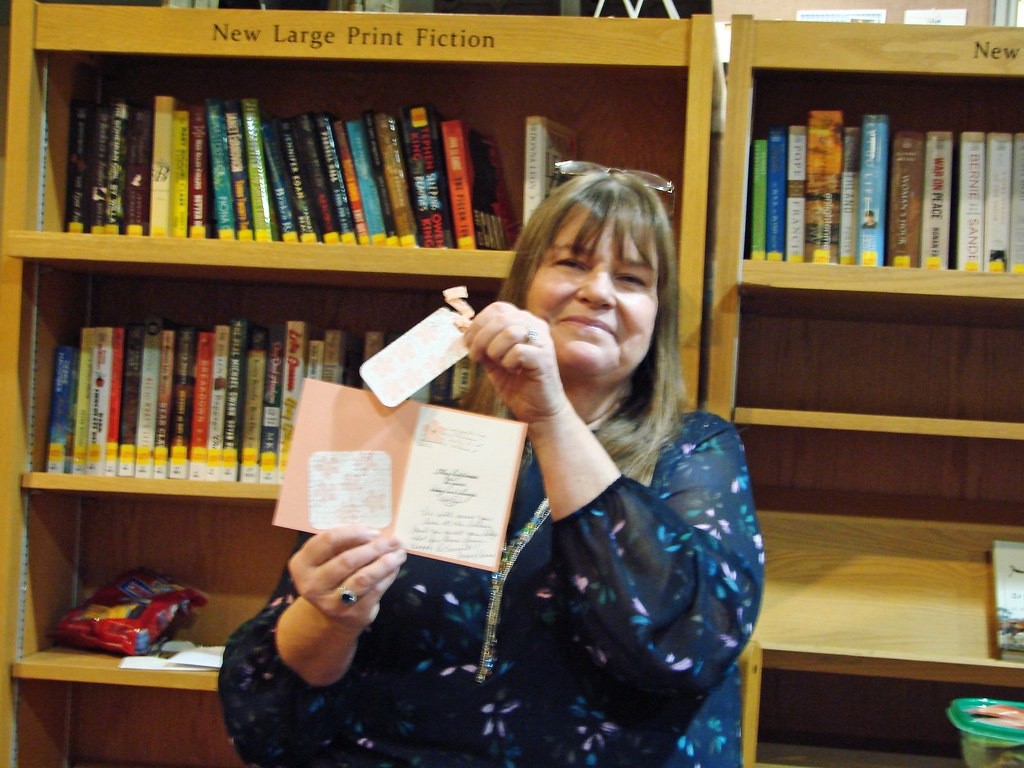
751 100 1024 273
64 92 582 253
987 539 1024 660
45 320 487 477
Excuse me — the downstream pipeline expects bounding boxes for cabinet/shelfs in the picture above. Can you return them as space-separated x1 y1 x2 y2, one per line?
708 13 1024 768
0 0 714 768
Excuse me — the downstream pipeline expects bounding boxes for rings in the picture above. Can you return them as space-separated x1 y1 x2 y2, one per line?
524 323 538 343
337 585 359 606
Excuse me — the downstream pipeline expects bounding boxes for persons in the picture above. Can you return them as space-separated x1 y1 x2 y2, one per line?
216 160 765 768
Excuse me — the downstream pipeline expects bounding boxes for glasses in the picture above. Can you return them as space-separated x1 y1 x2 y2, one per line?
544 161 675 222
